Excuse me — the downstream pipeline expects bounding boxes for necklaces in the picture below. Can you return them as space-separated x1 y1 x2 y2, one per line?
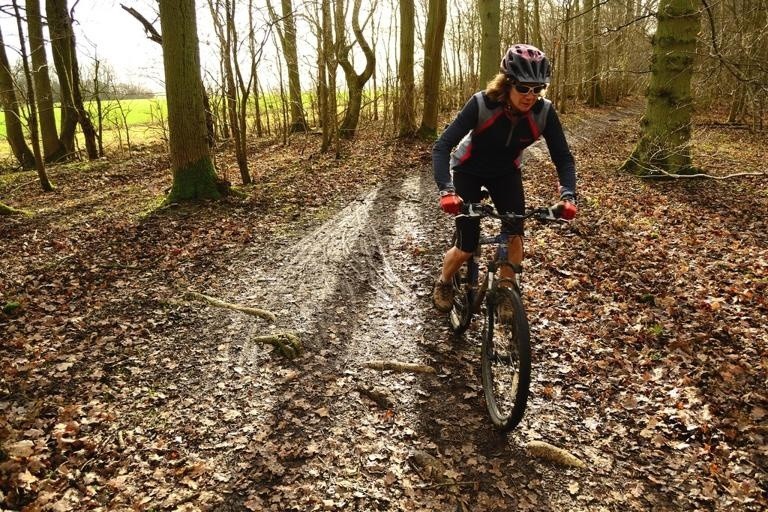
507 103 520 117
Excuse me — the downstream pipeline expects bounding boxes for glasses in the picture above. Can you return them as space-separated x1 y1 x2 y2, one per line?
511 84 544 95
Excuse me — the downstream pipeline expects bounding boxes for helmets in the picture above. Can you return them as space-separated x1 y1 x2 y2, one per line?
501 44 551 83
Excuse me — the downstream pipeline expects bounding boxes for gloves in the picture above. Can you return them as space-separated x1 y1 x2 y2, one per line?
439 189 463 214
554 192 578 220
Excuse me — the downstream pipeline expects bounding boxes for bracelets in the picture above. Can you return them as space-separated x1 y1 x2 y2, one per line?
438 188 457 197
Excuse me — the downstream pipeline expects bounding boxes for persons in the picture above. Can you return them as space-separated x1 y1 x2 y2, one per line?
430 44 577 323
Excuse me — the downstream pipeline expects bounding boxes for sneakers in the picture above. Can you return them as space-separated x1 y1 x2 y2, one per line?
497 295 515 322
432 280 453 312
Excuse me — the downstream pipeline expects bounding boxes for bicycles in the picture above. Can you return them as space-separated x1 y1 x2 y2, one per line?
436 185 581 436
